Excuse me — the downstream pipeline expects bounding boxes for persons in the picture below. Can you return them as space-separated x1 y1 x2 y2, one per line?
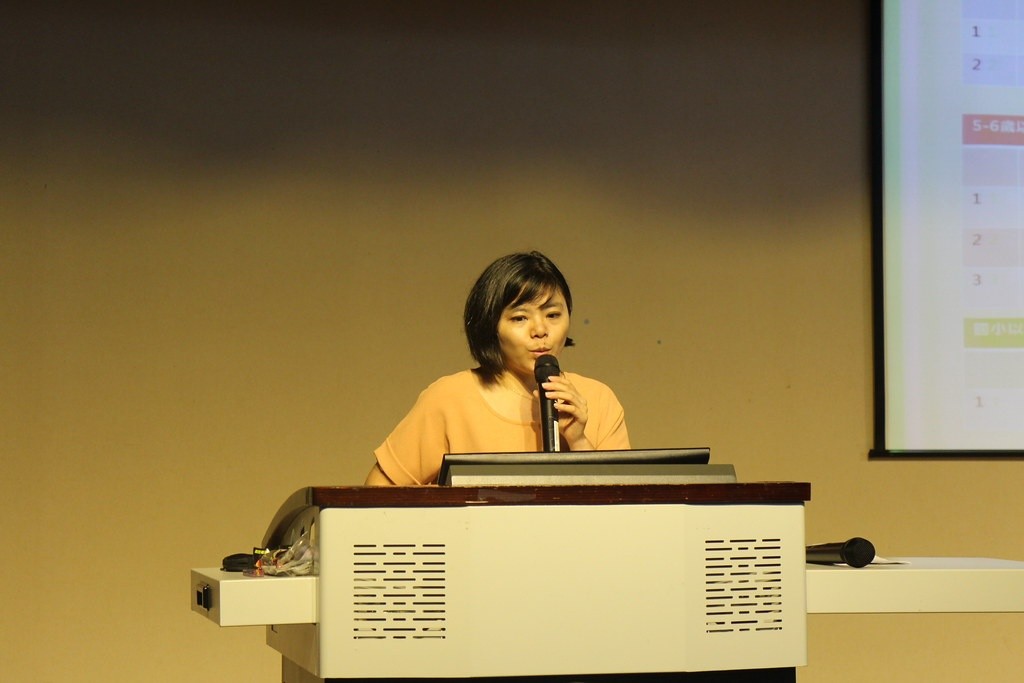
365 251 630 486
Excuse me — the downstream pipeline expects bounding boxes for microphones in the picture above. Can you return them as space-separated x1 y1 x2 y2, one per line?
806 537 875 568
534 354 561 451
